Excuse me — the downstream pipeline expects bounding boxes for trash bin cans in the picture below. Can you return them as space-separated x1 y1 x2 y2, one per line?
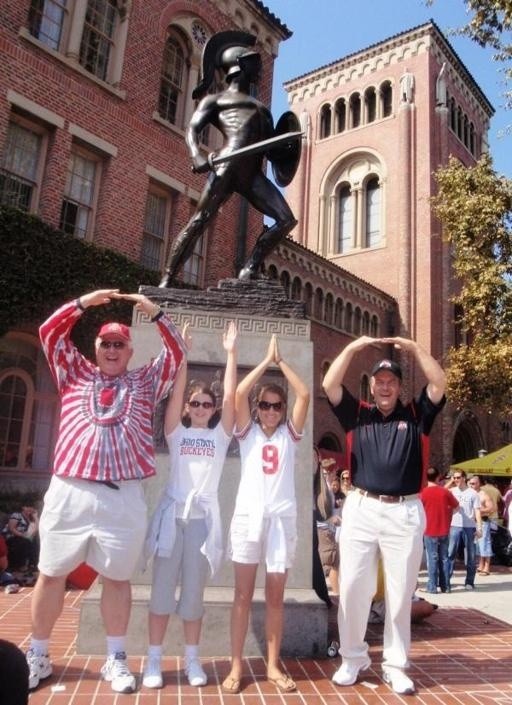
149 303 161 318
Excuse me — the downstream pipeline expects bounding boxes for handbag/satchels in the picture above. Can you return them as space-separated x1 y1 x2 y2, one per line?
275 357 284 368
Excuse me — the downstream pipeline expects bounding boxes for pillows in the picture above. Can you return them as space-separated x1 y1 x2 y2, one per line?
223 46 262 84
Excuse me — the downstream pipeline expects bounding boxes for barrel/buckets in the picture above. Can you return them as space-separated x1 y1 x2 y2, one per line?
222 675 241 694
477 568 482 572
265 673 297 692
479 570 490 576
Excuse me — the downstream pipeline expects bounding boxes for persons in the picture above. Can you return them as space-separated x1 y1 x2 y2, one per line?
2 500 39 583
367 551 438 624
331 477 347 511
320 457 342 595
321 334 449 695
24 288 189 693
443 469 484 590
339 466 354 494
141 319 239 690
418 467 459 594
155 29 306 289
468 476 495 575
219 333 311 694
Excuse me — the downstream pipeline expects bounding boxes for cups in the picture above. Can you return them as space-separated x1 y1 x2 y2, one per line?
454 475 465 483
190 400 213 409
259 400 285 411
340 478 351 484
98 341 130 350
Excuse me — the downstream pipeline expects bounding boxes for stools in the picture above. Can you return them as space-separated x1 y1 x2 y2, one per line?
358 487 409 503
92 480 120 490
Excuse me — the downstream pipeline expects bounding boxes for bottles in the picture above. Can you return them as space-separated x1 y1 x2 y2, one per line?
98 322 131 340
372 359 402 379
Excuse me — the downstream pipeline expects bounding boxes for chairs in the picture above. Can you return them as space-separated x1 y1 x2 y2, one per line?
143 654 164 688
465 584 474 591
383 667 416 694
332 655 373 686
444 590 451 593
26 650 54 691
419 588 437 594
184 655 208 686
101 651 137 693
